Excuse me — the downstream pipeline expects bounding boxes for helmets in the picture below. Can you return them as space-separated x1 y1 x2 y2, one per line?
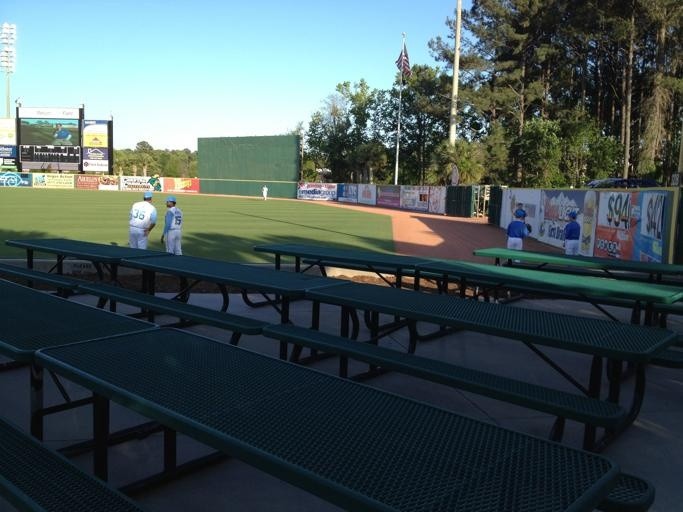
514 209 526 218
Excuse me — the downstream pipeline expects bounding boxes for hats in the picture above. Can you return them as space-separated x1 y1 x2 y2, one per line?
166 196 176 202
144 191 153 198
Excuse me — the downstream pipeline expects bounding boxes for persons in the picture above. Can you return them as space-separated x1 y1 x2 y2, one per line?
563 212 580 254
513 203 525 225
160 196 183 256
128 192 156 249
53 123 71 142
506 209 531 262
262 185 268 200
